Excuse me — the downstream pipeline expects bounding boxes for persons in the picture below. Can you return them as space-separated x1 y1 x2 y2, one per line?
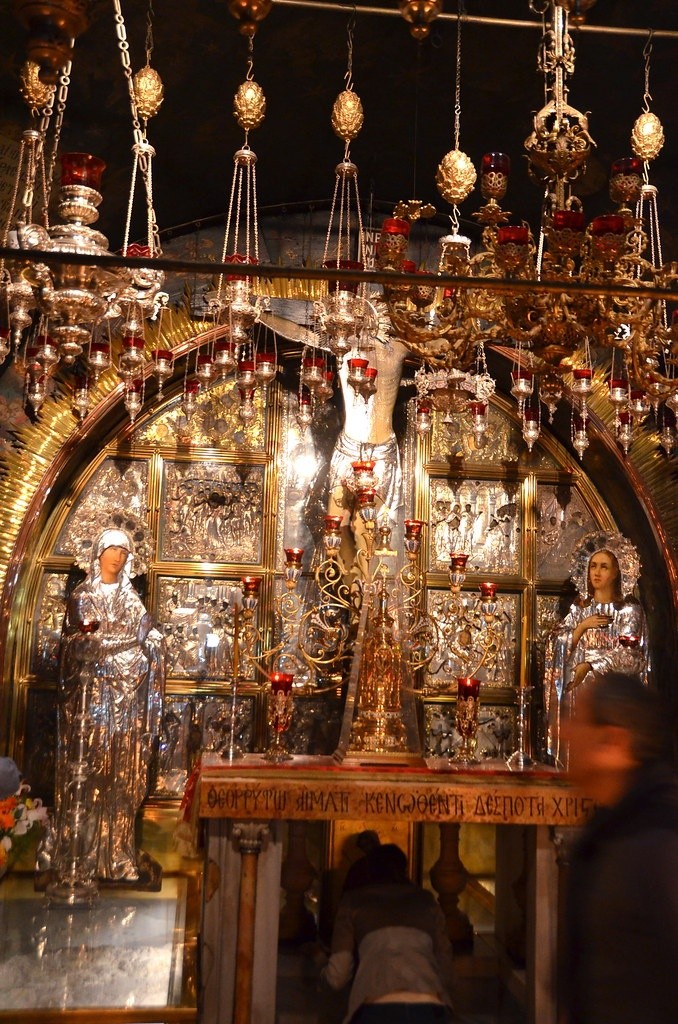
231 298 412 584
557 672 678 1024
310 844 458 1024
35 528 168 881
543 528 651 771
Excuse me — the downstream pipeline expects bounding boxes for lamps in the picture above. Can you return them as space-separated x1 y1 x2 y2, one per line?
2 38 678 458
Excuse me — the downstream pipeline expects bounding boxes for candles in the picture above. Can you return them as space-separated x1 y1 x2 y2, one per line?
238 453 499 695
